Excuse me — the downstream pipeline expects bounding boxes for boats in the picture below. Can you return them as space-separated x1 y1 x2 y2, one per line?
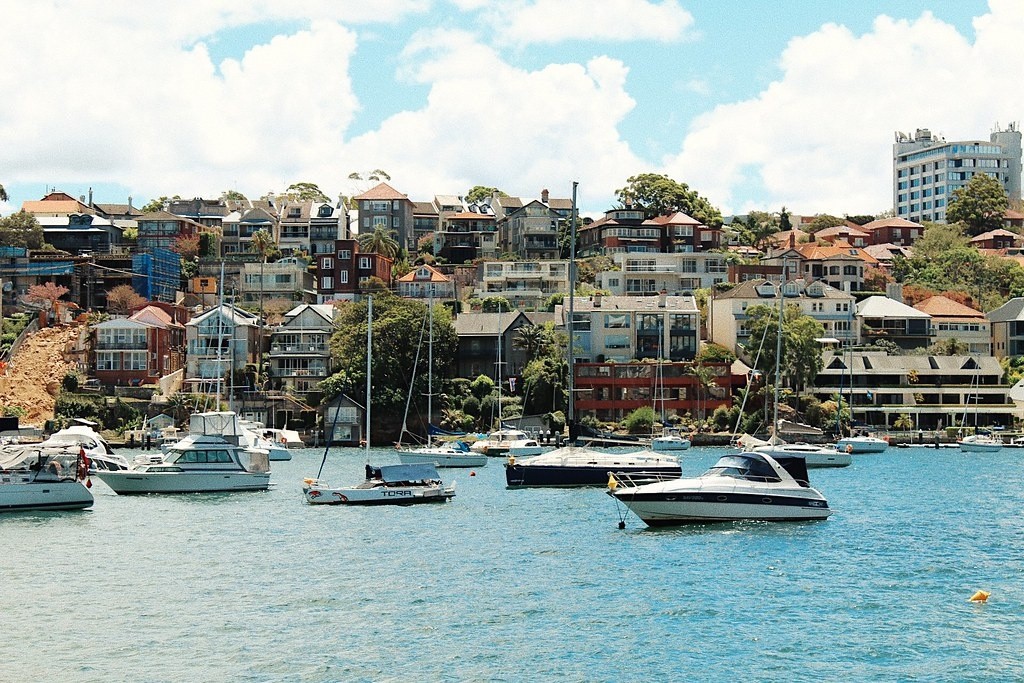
27 426 130 474
605 451 833 526
0 450 94 512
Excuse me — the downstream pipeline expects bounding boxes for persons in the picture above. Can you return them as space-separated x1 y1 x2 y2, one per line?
254 382 259 391
241 381 248 391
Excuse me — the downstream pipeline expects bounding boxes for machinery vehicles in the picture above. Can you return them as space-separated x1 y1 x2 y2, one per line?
47 300 80 323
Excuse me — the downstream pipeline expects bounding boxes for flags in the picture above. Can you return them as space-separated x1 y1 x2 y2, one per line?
509 378 516 392
79 449 92 488
867 391 872 400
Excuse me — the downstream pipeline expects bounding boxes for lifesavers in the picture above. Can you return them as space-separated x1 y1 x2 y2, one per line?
281 437 287 445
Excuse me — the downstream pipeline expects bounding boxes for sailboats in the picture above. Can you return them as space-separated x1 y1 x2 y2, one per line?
393 291 488 468
469 301 542 457
652 321 691 449
302 296 456 504
837 300 889 453
957 353 1003 452
728 257 853 468
94 261 271 495
161 281 292 461
502 182 683 490
